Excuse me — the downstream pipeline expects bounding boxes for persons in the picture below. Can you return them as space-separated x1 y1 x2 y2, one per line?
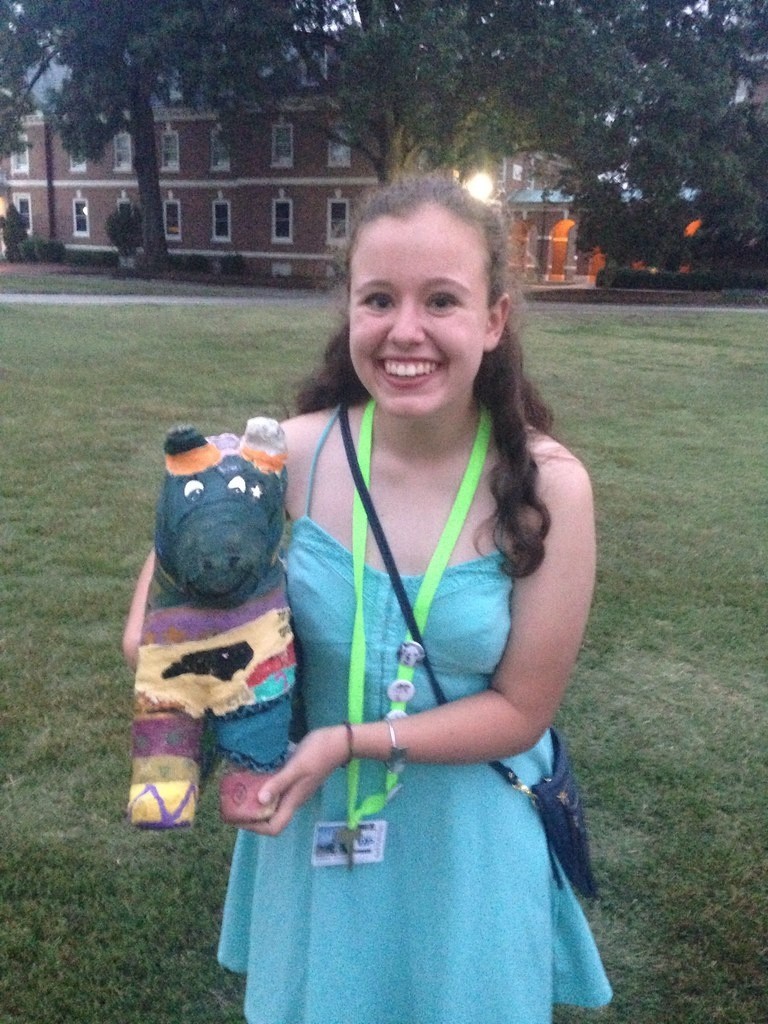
122 176 613 1024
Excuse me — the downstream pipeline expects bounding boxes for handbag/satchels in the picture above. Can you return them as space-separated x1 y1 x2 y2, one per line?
529 728 598 898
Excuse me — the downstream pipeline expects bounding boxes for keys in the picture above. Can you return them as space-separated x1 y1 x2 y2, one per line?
340 826 359 873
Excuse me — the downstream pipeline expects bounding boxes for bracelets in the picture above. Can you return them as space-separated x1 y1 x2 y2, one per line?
339 718 358 772
382 718 410 776
